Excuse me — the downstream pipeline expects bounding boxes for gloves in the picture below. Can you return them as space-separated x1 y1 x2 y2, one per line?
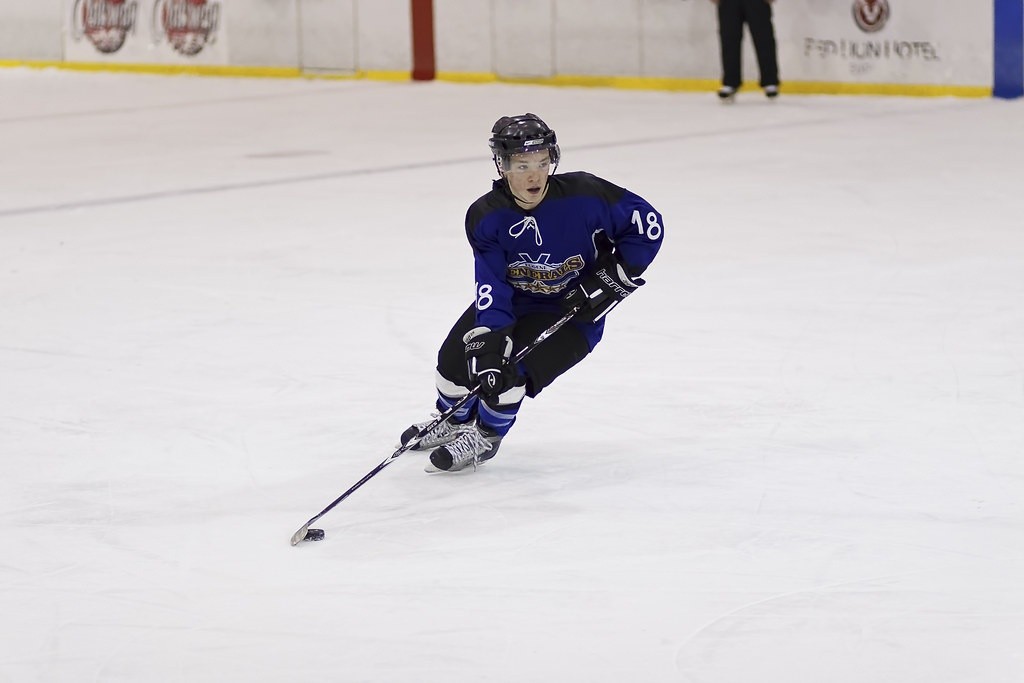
462 327 525 407
563 259 647 323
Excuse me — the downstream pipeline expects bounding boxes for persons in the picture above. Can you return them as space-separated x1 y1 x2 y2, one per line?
394 111 665 475
712 0 781 106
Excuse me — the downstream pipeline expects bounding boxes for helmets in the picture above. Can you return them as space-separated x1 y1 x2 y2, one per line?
488 112 560 167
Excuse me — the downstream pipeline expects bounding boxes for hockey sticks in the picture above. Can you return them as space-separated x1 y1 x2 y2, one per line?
291 304 582 547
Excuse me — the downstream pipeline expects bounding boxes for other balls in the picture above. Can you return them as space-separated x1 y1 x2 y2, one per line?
303 529 325 542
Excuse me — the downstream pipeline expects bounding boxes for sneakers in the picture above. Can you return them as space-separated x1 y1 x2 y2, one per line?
718 86 739 105
395 400 478 451
765 85 780 101
424 416 516 473
